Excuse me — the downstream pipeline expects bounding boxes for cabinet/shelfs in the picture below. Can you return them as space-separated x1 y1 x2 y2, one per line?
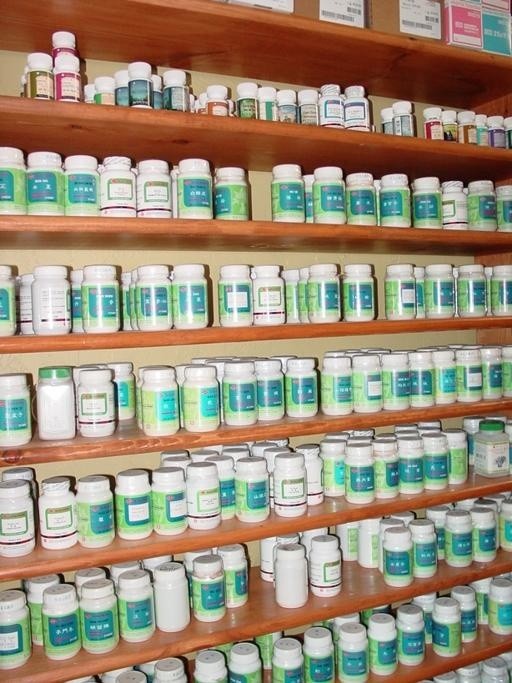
0 0 511 682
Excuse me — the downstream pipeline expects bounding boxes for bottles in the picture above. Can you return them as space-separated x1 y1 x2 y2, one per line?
2 31 512 683
